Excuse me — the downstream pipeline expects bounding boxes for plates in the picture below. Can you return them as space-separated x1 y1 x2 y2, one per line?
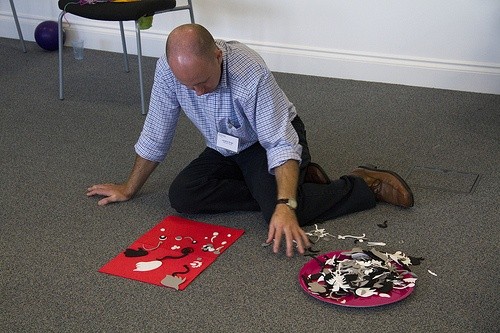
298 250 413 307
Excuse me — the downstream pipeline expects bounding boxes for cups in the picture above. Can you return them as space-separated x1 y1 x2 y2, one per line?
72 39 84 59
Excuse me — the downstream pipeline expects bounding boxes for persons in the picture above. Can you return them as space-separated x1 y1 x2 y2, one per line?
85 24 415 257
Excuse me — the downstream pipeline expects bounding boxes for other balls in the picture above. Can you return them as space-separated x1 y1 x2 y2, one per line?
34 20 67 52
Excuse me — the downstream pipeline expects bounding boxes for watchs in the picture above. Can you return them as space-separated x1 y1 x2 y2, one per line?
276 196 298 209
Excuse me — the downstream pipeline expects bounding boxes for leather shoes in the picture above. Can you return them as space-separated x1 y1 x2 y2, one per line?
304 162 330 184
350 165 414 208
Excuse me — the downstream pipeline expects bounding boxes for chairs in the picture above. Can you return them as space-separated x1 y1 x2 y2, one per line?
56 0 198 115
